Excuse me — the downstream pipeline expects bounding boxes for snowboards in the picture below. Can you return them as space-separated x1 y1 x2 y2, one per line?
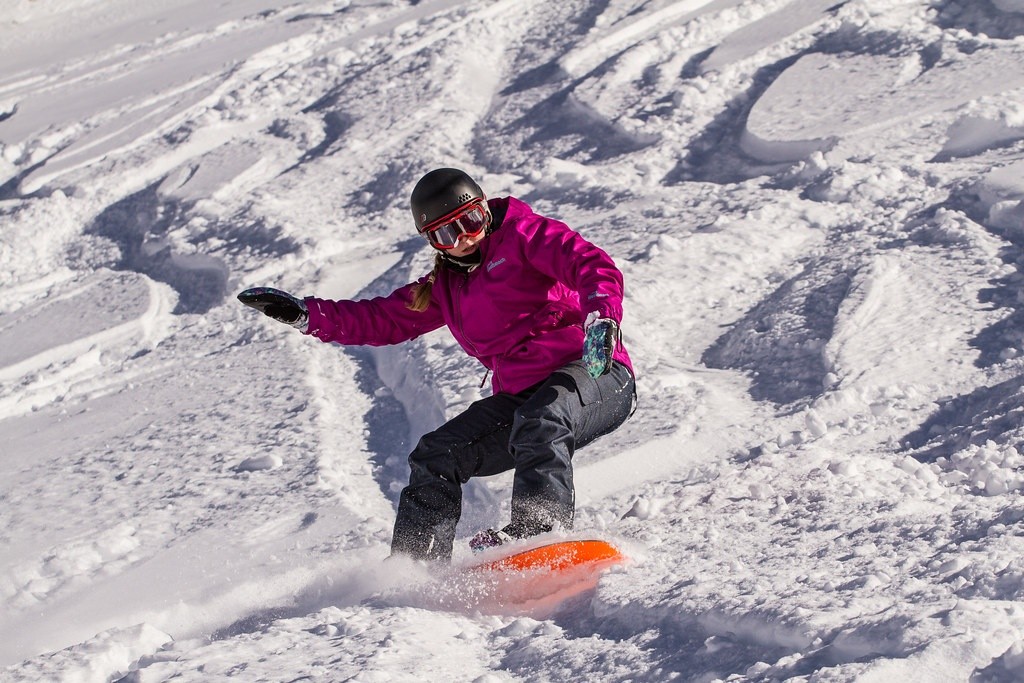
361 538 629 610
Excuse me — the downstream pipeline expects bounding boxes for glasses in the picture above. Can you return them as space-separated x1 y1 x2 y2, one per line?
428 203 489 249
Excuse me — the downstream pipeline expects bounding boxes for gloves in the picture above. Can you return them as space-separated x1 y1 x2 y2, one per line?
582 318 620 377
237 287 309 329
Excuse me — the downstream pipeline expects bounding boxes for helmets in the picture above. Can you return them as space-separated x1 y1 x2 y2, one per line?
411 168 482 233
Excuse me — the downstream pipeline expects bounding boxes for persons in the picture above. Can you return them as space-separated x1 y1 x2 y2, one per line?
237 167 639 566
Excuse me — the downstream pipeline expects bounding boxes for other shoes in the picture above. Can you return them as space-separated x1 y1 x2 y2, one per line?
469 525 523 549
